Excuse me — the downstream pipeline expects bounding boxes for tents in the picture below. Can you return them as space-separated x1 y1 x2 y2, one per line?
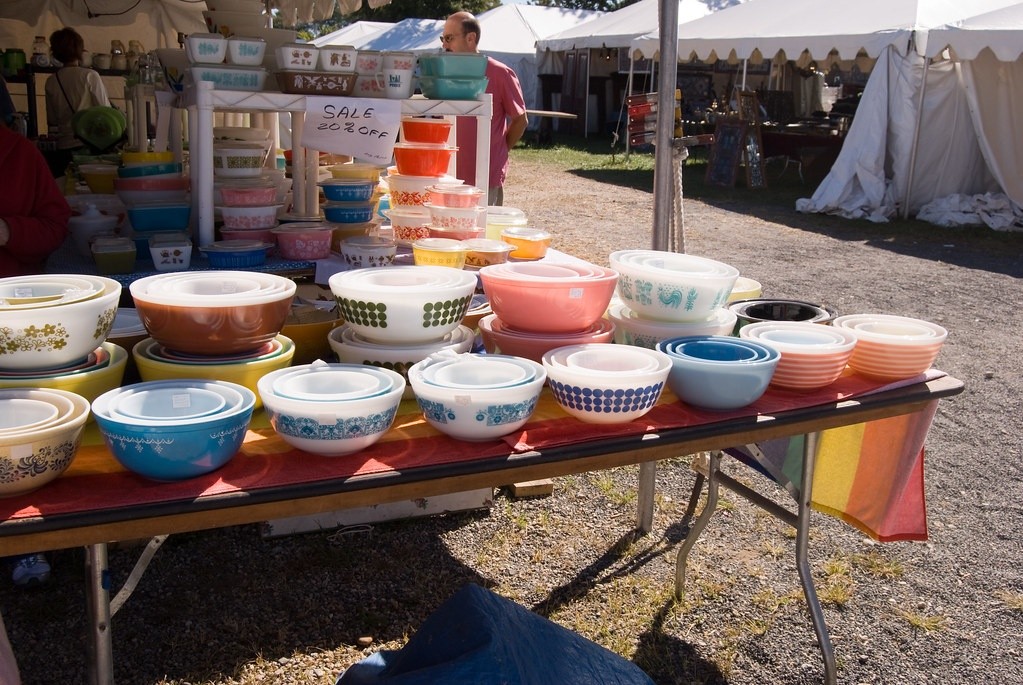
0 0 679 531
306 0 1023 230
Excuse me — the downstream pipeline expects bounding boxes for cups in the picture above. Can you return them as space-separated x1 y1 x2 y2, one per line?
67 204 119 261
375 68 414 99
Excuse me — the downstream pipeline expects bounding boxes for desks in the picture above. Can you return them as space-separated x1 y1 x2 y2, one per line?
25 65 130 137
759 128 844 186
0 364 967 685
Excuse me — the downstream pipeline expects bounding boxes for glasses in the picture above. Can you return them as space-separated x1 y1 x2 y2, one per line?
440 32 467 42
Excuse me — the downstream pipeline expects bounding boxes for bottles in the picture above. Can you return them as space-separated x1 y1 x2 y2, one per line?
0 34 62 74
32 135 56 151
12 112 30 138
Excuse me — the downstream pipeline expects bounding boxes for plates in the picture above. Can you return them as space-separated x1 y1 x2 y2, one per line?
71 106 128 146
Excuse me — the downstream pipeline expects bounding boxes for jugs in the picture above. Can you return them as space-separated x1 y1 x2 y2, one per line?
79 40 145 70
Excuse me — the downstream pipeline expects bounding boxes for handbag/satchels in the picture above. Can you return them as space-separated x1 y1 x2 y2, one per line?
825 63 843 87
845 64 867 87
766 61 796 123
832 93 862 114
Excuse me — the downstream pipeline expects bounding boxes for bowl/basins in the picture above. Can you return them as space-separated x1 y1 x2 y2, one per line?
91 237 136 274
147 233 193 271
184 4 546 263
0 263 947 496
155 47 196 95
56 150 184 256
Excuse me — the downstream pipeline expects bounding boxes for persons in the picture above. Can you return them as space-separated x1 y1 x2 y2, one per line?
440 11 529 207
45 27 112 177
0 76 72 278
